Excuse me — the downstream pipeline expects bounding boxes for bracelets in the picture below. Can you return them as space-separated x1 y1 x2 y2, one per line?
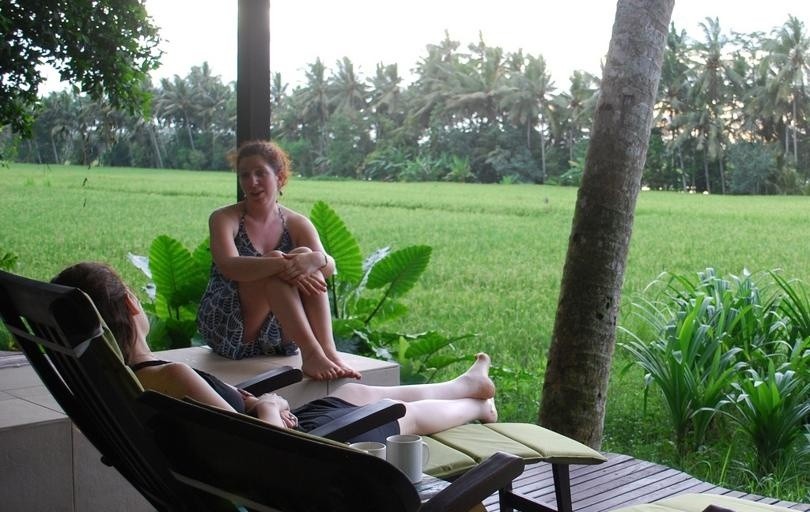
236 385 253 399
322 251 329 268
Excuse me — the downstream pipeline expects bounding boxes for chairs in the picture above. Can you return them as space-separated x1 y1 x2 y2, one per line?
140 388 806 512
0 272 603 512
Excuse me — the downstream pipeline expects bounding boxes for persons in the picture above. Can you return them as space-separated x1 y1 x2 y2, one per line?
196 139 362 382
47 260 499 452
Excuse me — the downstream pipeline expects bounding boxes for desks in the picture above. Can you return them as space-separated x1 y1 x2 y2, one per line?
374 464 456 504
